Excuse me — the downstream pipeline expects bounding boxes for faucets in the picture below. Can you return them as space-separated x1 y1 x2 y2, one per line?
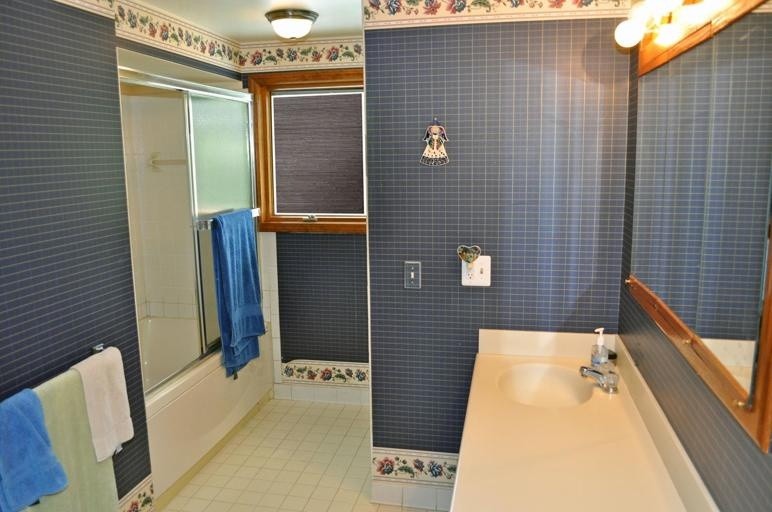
578 365 605 384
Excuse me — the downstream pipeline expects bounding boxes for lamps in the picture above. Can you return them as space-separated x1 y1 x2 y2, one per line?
265 8 319 39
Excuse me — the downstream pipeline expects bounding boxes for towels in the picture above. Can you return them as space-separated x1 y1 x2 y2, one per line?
0 346 135 512
208 208 265 377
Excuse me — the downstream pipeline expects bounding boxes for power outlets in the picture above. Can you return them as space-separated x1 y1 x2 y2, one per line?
461 256 491 286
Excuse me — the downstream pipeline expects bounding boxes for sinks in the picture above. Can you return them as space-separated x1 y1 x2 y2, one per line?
497 362 594 410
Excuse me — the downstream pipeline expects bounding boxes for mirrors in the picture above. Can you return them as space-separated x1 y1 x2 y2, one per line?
624 3 769 450
